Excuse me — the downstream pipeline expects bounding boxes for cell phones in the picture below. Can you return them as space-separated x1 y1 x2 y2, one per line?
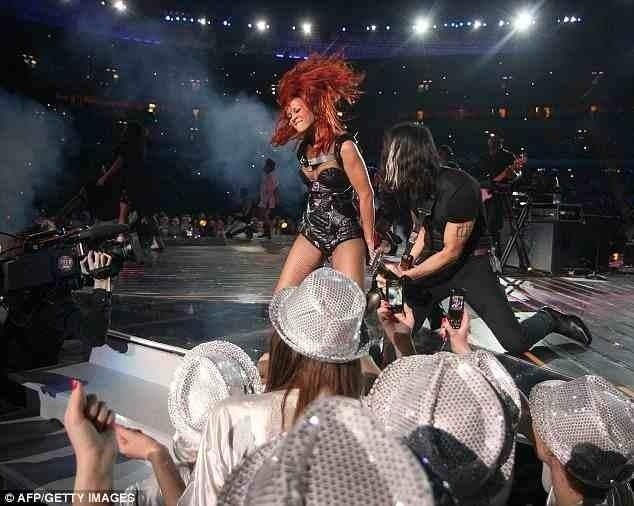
448 289 466 328
385 279 404 314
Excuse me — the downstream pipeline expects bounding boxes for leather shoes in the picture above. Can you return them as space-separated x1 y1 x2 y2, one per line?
540 306 592 346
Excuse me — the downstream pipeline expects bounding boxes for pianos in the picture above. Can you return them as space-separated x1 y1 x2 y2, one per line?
482 178 535 193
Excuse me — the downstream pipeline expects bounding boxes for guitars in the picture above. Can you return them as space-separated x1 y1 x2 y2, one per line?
373 254 414 314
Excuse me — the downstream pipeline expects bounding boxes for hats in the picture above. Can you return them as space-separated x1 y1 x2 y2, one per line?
218 350 634 502
169 343 262 447
267 265 372 366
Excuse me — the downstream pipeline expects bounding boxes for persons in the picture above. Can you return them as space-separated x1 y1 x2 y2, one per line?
269 46 378 315
0 115 634 506
